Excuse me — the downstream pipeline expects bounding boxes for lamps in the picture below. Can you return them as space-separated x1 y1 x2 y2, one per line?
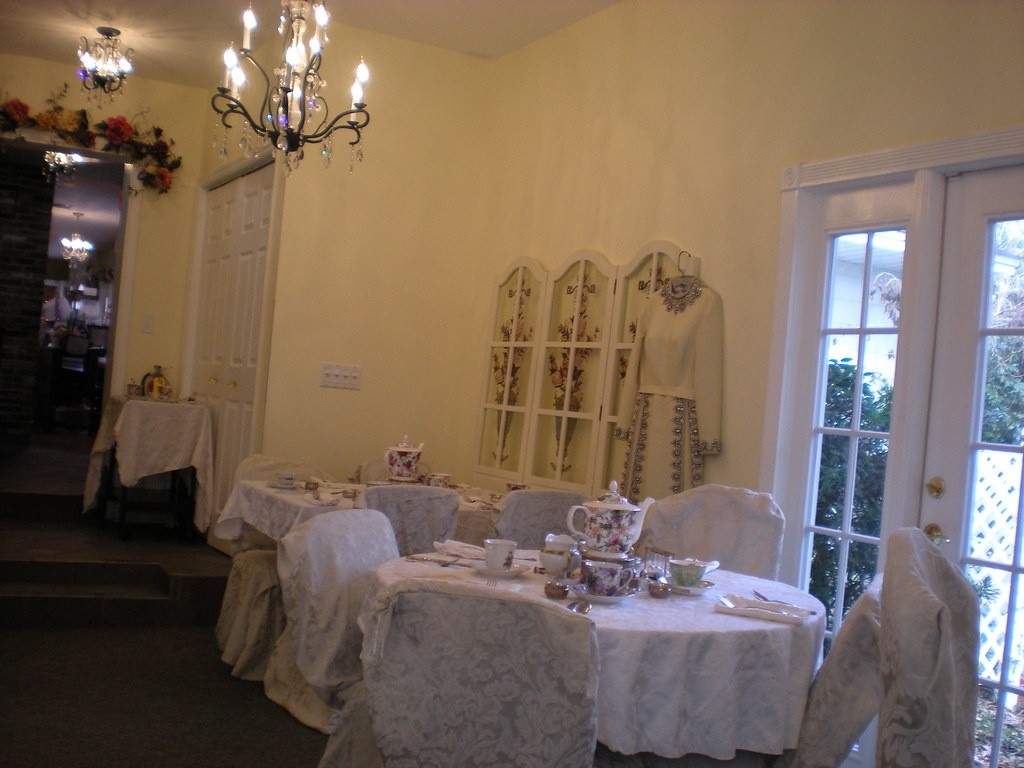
61 213 93 263
76 25 136 106
211 0 369 175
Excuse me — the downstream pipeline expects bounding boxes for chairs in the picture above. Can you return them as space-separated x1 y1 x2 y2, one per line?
353 452 413 483
493 490 583 551
279 509 400 689
648 484 786 580
355 485 459 558
762 571 881 767
876 526 980 768
359 576 600 767
217 453 322 638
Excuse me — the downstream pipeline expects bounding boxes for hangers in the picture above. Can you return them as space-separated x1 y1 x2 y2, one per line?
661 250 718 301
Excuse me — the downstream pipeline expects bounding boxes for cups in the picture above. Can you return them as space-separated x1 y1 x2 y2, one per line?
427 473 452 488
366 481 391 486
277 473 296 484
668 560 707 587
305 482 318 490
168 387 177 402
584 561 633 597
485 539 517 570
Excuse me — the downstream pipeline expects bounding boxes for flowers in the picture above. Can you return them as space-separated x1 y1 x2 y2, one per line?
493 306 531 462
619 318 646 380
0 84 182 196
549 294 599 472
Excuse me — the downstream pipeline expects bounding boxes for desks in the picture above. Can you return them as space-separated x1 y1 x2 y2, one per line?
214 479 505 548
82 395 212 539
355 548 828 761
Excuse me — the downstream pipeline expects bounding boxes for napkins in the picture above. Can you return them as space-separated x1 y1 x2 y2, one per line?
463 495 501 511
680 555 719 574
434 538 485 556
716 592 807 625
304 491 339 507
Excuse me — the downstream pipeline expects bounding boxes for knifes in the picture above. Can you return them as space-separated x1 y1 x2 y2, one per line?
408 556 472 567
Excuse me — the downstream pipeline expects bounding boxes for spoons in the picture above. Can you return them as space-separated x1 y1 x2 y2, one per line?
752 591 817 615
717 595 789 616
404 558 460 567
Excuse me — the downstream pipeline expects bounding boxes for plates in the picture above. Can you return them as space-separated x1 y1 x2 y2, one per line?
268 483 297 489
124 394 146 401
714 594 810 625
658 577 716 597
570 584 636 604
478 564 530 578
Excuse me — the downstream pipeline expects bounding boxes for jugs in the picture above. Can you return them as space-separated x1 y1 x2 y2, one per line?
539 534 583 584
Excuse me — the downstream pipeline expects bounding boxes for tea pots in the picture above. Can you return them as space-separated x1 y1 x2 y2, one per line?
385 434 424 481
567 480 656 559
